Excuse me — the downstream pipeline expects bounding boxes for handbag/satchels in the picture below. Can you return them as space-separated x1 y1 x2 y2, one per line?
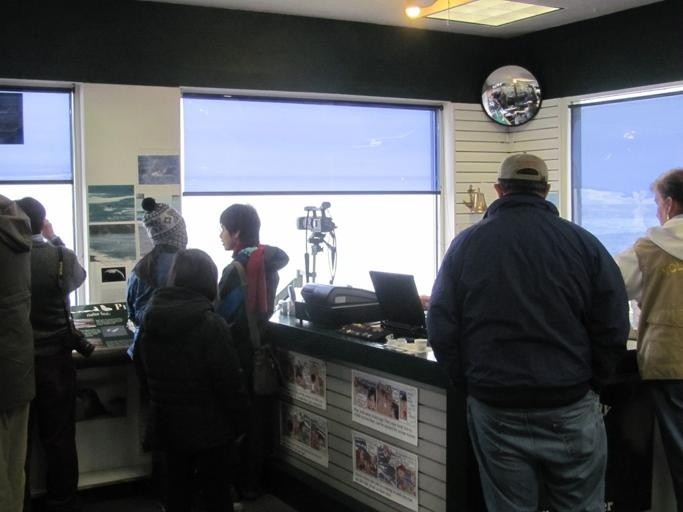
252 348 288 398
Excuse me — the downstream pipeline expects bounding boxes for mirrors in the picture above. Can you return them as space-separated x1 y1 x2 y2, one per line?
480 63 543 127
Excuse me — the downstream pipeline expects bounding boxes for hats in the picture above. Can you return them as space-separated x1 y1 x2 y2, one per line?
497 152 549 182
141 198 187 250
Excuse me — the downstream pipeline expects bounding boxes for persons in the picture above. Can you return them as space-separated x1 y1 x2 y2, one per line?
125 196 188 359
16 197 86 512
425 150 629 512
610 168 683 512
0 193 37 512
217 203 290 503
133 248 254 512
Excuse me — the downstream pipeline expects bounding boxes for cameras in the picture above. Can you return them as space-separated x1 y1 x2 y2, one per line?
297 202 335 232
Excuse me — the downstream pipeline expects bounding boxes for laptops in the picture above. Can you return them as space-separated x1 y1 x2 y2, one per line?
368 271 428 338
